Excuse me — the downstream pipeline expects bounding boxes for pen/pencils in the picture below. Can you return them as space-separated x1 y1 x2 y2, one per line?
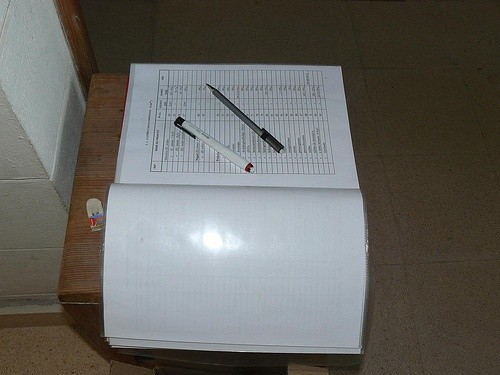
206 83 285 155
173 116 256 174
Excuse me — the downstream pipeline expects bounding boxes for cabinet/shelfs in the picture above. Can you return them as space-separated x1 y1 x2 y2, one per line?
54 73 288 373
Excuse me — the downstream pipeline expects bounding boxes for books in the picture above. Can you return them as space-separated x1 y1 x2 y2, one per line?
101 63 371 354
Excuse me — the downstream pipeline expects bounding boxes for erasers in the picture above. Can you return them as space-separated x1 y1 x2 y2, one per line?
86 198 105 232
